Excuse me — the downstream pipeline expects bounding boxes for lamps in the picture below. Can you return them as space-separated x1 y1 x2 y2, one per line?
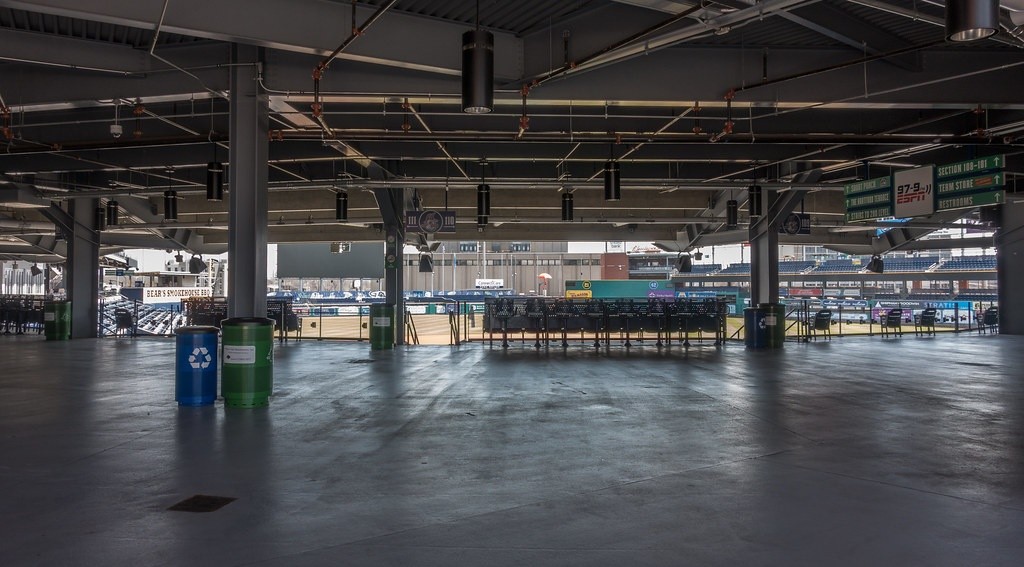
748 166 762 218
477 161 491 217
944 0 1000 43
109 98 123 138
207 142 223 201
562 188 573 221
727 192 737 227
164 173 178 221
95 202 106 232
478 216 488 225
107 197 118 226
604 144 621 201
461 0 495 114
336 190 348 221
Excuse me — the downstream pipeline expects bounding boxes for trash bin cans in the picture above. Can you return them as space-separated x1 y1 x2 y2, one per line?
172 324 220 407
757 301 786 349
221 316 274 410
742 307 771 351
43 299 73 341
369 302 395 350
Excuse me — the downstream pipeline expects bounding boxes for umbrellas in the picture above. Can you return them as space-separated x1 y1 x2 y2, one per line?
538 273 552 286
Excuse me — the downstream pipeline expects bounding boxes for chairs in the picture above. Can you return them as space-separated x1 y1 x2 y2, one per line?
977 306 999 334
880 308 902 338
181 295 303 342
0 294 54 335
113 308 138 339
913 307 936 337
482 296 727 349
809 310 831 341
